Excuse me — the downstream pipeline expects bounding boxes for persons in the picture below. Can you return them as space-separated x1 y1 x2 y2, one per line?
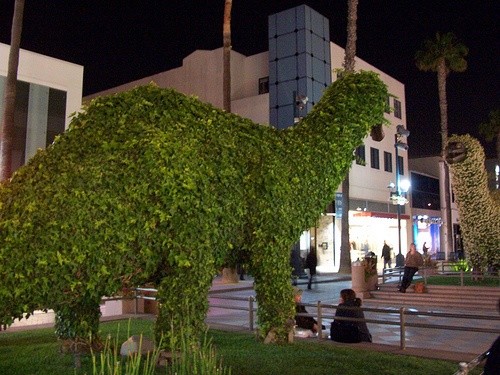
330 289 373 343
364 240 369 253
423 242 428 253
239 269 245 280
381 239 393 272
293 286 326 332
484 296 500 375
399 243 423 292
291 250 299 286
351 241 357 250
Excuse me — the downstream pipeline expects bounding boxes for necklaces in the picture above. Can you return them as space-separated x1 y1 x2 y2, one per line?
306 246 319 288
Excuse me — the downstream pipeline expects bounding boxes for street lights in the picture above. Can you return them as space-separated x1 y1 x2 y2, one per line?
388 124 409 268
